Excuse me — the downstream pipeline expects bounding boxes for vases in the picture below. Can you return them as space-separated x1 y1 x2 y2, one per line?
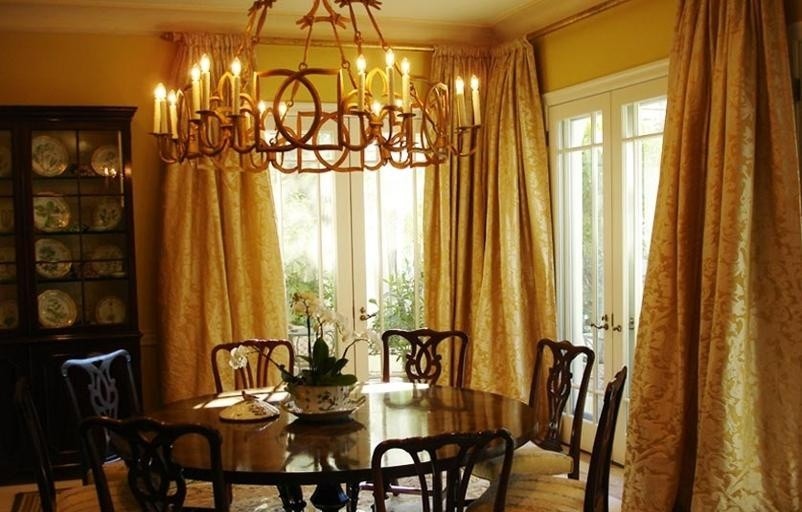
289 386 350 411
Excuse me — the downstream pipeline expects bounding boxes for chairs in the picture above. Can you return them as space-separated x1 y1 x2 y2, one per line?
14 377 144 511
60 348 169 511
349 329 469 512
370 429 513 512
78 416 229 512
463 362 629 511
459 338 595 511
212 341 303 512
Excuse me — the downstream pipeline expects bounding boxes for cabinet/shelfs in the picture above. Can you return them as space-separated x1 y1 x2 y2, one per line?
0 104 138 487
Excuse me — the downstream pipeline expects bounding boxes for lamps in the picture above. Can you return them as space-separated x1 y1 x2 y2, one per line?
148 0 480 173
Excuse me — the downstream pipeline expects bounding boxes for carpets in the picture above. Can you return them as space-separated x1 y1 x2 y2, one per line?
9 455 622 512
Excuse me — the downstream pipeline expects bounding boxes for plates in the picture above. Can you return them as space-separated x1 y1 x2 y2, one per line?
282 416 366 435
0 247 18 282
87 195 123 230
0 143 12 176
35 239 73 278
89 243 124 276
91 144 121 176
1 295 18 329
33 136 71 177
38 288 78 330
1 192 14 234
94 296 126 325
32 191 71 232
281 397 365 419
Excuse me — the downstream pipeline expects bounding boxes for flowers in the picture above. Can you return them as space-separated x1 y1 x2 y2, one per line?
228 290 383 394
